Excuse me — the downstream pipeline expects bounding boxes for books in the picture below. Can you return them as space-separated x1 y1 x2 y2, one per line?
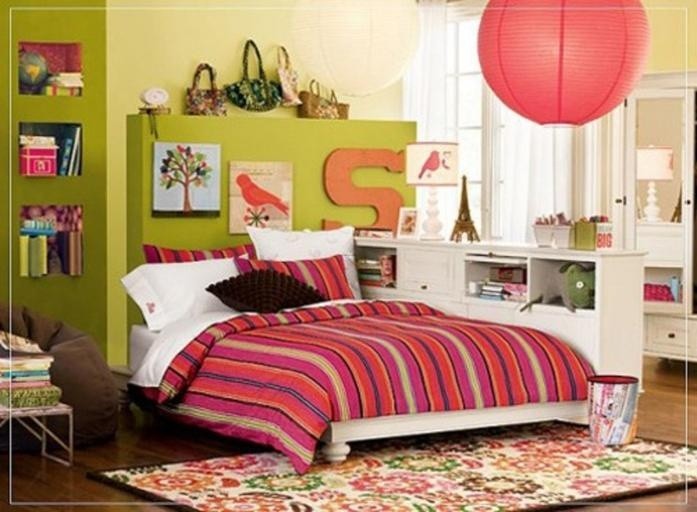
59 126 80 176
0 330 55 388
480 281 527 302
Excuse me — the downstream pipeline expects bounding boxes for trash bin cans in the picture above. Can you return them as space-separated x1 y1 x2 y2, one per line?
587 374 639 445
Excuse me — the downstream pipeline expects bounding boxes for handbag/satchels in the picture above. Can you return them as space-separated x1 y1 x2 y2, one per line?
276 69 303 108
296 79 349 120
228 79 282 111
186 87 227 116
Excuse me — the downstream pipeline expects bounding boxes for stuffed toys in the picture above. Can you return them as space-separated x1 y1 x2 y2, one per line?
561 264 595 312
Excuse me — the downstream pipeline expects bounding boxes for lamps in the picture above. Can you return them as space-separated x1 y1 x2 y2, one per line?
405 142 459 242
636 145 674 222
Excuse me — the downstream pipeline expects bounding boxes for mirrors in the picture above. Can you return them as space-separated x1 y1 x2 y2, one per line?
630 89 687 227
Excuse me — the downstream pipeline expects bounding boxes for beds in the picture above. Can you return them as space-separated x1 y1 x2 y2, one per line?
129 297 596 465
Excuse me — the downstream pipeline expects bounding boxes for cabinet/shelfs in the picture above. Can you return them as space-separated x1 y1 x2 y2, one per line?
612 72 696 365
352 235 648 398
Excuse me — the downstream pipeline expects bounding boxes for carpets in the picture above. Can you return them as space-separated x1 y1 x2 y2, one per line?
86 420 696 511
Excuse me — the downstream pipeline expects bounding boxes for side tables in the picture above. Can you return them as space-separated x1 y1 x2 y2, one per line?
2 402 74 468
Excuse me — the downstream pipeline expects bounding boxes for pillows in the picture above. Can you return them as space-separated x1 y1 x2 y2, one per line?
120 226 362 331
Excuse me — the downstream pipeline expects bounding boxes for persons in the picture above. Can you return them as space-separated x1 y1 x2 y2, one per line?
380 254 396 288
